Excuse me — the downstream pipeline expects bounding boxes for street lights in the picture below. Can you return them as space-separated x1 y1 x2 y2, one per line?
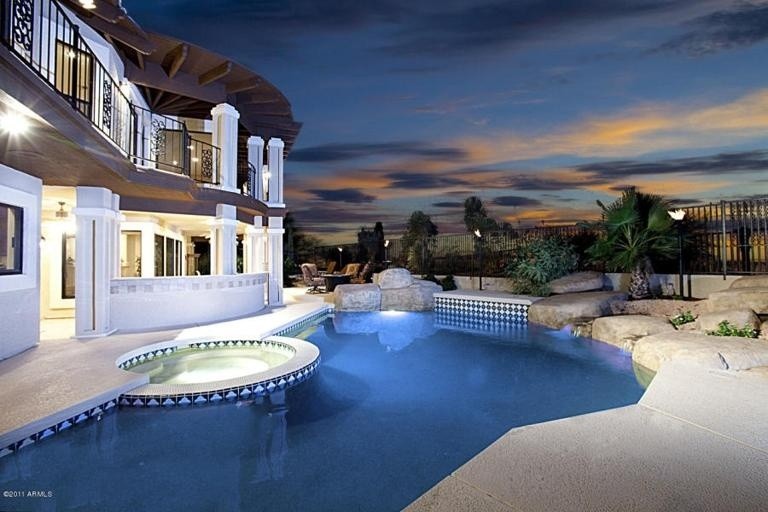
335 245 343 271
382 239 391 270
470 227 484 293
667 207 689 299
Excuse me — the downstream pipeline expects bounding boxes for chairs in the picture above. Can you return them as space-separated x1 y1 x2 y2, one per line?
300 261 376 294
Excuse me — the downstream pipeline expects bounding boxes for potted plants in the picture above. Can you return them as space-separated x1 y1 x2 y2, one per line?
579 186 698 299
